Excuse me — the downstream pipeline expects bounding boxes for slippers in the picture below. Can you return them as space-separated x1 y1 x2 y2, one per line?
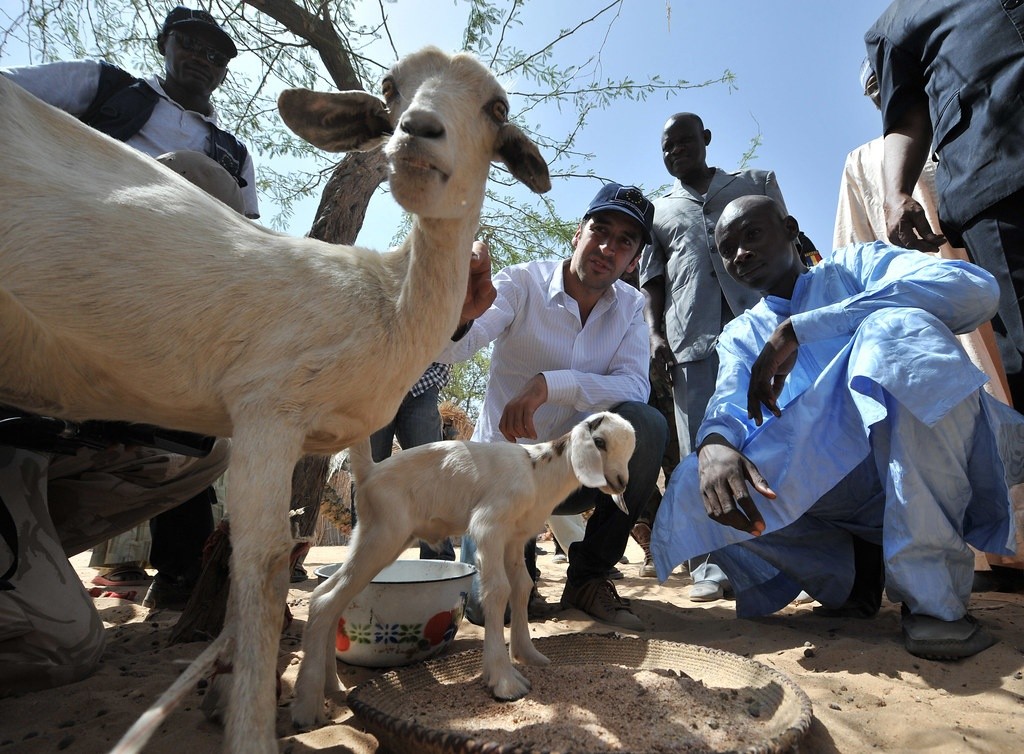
92 568 155 586
291 570 308 582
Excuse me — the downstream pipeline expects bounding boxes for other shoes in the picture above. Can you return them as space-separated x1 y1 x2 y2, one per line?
607 567 623 579
689 581 723 601
813 601 866 619
534 545 547 555
552 554 567 564
900 606 979 656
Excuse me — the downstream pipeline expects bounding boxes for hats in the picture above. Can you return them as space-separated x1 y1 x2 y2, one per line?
162 7 237 58
861 59 876 96
584 183 655 246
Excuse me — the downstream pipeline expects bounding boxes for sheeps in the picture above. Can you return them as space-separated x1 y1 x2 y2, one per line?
290 412 638 728
0 43 553 754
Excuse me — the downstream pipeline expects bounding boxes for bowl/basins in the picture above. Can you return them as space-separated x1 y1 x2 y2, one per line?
314 559 478 667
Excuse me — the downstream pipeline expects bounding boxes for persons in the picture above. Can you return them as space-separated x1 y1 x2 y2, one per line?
0 6 259 611
370 363 456 561
832 54 1008 404
0 419 232 695
435 182 668 632
863 0 1024 413
640 112 788 459
651 195 1018 659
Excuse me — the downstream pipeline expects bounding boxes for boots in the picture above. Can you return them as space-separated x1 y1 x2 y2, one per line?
630 524 658 577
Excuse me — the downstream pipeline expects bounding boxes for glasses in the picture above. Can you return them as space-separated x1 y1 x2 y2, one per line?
166 31 230 67
866 81 880 98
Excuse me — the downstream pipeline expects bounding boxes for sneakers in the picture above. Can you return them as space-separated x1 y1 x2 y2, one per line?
560 576 645 631
531 581 549 614
